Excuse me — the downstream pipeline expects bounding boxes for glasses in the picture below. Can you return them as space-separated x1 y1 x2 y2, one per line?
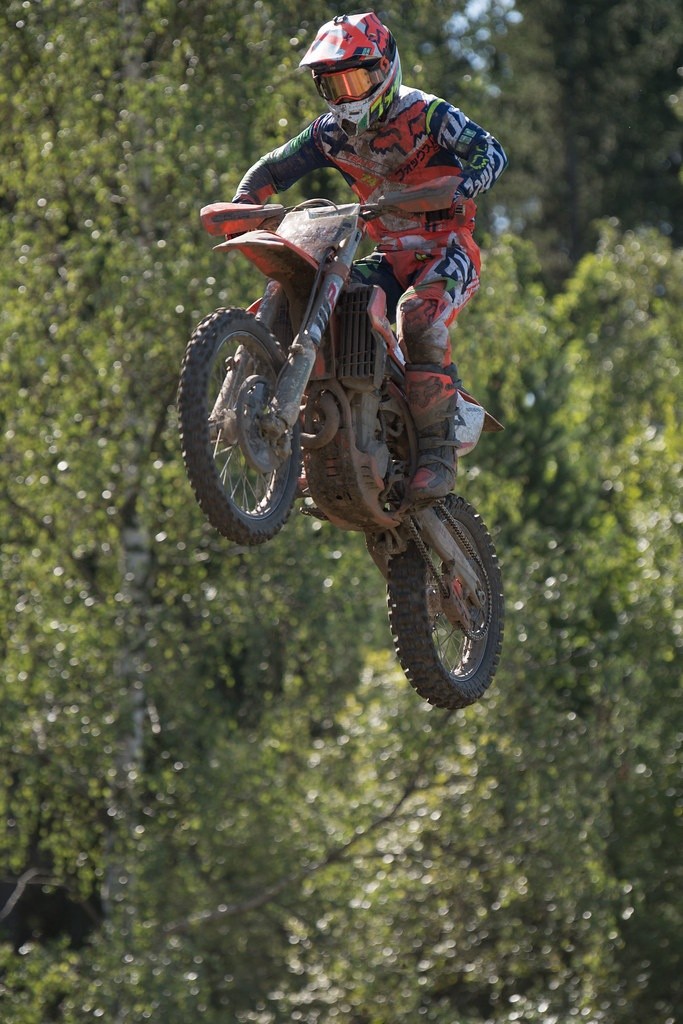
310 69 385 104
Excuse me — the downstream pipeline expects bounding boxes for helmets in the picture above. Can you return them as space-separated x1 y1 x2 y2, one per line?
297 12 401 138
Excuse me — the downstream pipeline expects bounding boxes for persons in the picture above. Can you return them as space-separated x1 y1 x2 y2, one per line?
224 9 506 503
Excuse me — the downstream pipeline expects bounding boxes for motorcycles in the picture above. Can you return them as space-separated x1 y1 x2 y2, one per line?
174 172 512 714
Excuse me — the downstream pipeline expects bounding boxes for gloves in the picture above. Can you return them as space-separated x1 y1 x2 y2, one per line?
416 186 469 233
223 205 257 240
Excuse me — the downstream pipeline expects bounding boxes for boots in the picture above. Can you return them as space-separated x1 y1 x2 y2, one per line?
404 363 460 500
